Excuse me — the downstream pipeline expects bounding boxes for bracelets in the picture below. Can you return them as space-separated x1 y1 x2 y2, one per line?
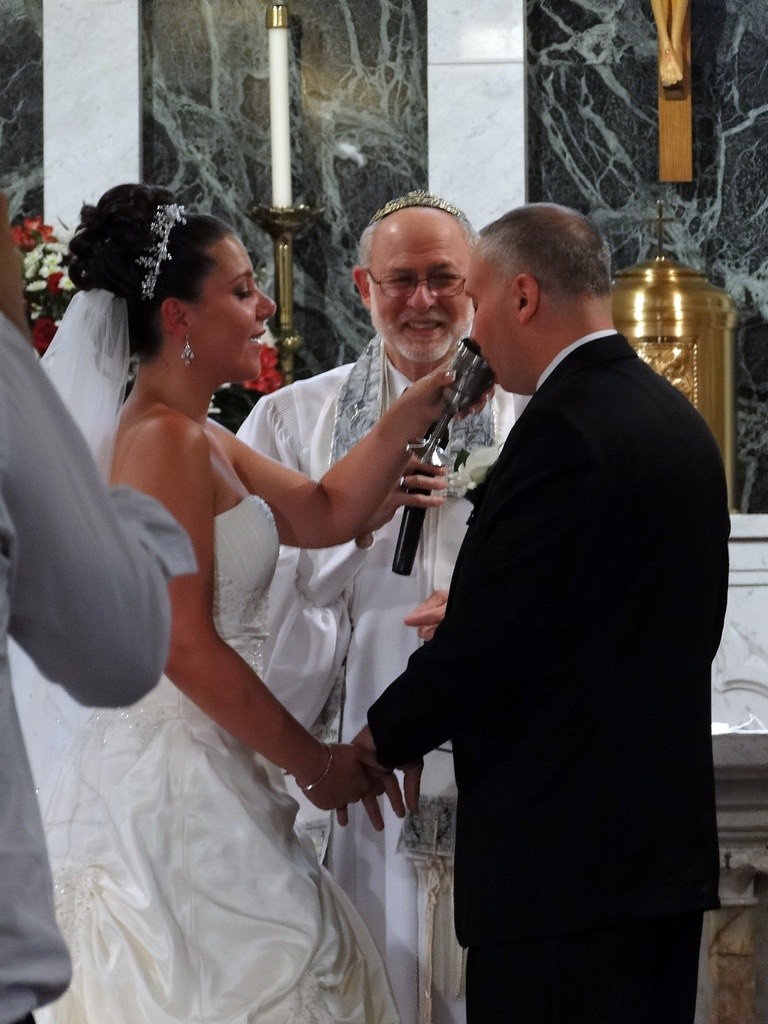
295 742 334 791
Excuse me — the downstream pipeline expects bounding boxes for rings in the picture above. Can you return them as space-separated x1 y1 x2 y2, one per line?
399 476 409 493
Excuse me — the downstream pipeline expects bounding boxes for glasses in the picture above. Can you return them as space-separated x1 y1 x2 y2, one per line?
366 268 466 299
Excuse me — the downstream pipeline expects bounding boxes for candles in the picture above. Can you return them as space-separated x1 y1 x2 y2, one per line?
263 1 293 210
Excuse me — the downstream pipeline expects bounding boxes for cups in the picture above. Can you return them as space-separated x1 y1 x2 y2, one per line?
406 338 496 468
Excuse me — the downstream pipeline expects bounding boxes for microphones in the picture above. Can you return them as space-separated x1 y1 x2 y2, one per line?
392 420 450 576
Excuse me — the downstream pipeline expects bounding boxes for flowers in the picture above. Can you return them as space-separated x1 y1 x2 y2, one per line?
11 214 77 354
462 445 498 485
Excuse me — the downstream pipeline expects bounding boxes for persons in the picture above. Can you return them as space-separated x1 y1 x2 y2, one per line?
234 189 534 1024
0 185 199 1024
335 202 731 1024
6 183 496 1023
